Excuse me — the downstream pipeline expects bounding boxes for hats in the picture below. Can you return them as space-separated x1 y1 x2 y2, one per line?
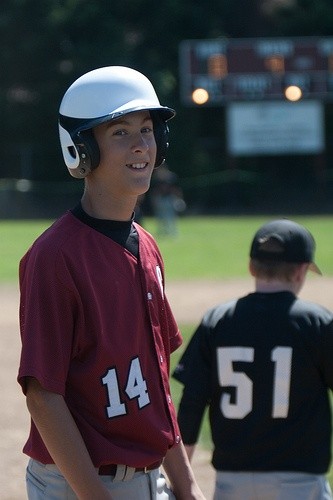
250 218 324 277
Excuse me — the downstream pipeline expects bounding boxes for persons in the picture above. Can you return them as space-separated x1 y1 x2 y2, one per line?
172 218 333 500
17 66 205 500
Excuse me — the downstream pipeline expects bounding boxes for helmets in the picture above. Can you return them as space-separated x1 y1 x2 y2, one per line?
58 64 175 178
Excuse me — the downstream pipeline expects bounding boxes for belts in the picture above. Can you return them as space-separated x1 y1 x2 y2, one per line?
95 465 160 477
